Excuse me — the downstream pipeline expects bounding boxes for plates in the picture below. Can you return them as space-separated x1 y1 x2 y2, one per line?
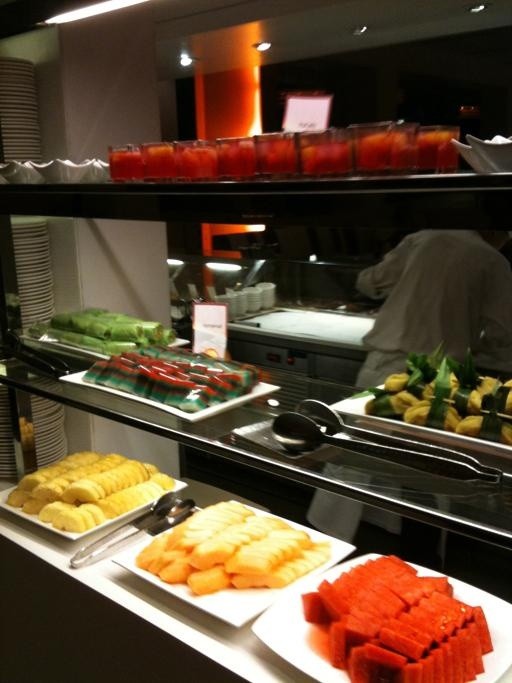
326 383 512 457
1 53 69 481
0 453 188 542
59 357 284 421
0 155 108 184
249 549 512 683
109 498 357 628
450 137 494 175
14 319 189 359
463 132 511 174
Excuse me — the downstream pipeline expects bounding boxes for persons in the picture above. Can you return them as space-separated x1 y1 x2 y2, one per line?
293 221 512 551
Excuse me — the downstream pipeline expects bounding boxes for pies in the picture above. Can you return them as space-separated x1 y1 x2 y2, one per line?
364 372 512 446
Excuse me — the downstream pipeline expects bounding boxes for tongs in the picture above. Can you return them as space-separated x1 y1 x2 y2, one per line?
70 492 195 568
272 399 512 485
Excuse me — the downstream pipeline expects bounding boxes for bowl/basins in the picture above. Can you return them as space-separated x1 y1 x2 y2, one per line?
216 281 276 319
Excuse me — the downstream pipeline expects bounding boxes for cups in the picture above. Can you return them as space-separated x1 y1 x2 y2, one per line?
108 120 460 182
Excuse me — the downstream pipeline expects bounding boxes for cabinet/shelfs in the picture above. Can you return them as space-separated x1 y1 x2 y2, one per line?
0 167 511 594
